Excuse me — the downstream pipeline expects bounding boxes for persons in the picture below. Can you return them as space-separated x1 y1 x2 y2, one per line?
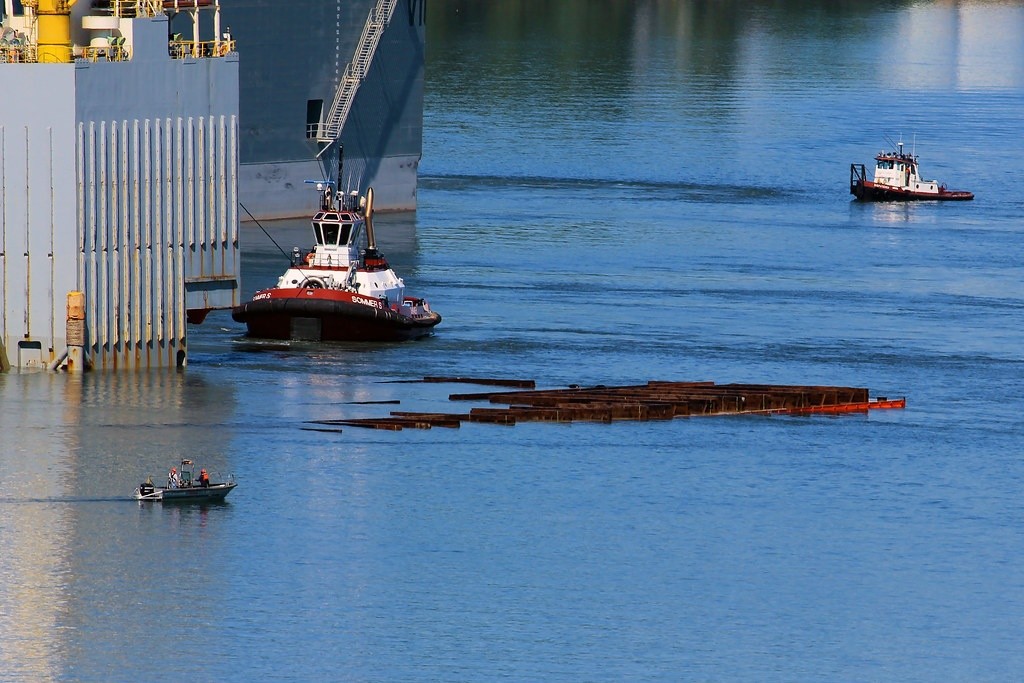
192 468 210 488
167 467 178 489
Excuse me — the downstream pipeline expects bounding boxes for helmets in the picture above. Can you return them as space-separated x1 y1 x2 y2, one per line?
171 467 176 473
201 468 206 473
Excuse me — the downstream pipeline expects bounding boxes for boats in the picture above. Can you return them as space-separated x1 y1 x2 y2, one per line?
224 141 442 350
848 128 976 203
127 457 238 504
